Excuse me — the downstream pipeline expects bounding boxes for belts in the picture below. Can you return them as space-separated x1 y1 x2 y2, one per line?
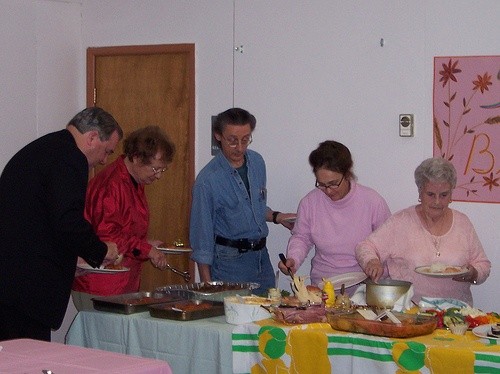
216 235 267 250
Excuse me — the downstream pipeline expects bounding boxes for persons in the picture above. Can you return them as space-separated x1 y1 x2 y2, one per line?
188 107 297 294
354 154 491 308
278 141 394 299
0 107 124 342
71 126 175 312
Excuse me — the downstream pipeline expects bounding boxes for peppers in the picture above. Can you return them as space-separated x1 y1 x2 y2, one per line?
405 307 500 329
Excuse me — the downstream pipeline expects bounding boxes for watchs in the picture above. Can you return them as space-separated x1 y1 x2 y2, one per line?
474 278 476 284
272 212 280 224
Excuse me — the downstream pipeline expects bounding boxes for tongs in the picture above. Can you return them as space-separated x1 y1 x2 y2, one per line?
164 263 191 282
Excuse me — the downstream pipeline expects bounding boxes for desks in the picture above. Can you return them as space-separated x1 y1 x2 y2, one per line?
64 310 500 374
0 338 173 374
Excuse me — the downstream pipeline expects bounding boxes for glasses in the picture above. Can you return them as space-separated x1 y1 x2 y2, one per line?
142 157 168 173
219 132 253 149
314 173 346 190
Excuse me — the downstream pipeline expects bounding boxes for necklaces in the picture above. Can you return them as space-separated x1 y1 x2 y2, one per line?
423 217 449 256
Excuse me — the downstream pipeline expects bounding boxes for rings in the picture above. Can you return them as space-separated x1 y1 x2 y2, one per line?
463 277 465 280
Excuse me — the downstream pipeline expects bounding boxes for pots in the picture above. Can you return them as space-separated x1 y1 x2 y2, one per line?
366 279 412 308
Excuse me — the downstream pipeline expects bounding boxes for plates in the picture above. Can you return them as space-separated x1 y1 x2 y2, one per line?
414 264 468 277
326 309 437 337
77 264 131 273
156 248 192 254
319 272 366 291
472 323 500 340
282 217 296 223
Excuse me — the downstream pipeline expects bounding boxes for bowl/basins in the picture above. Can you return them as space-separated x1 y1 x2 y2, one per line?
450 323 470 335
169 281 261 302
224 296 276 325
